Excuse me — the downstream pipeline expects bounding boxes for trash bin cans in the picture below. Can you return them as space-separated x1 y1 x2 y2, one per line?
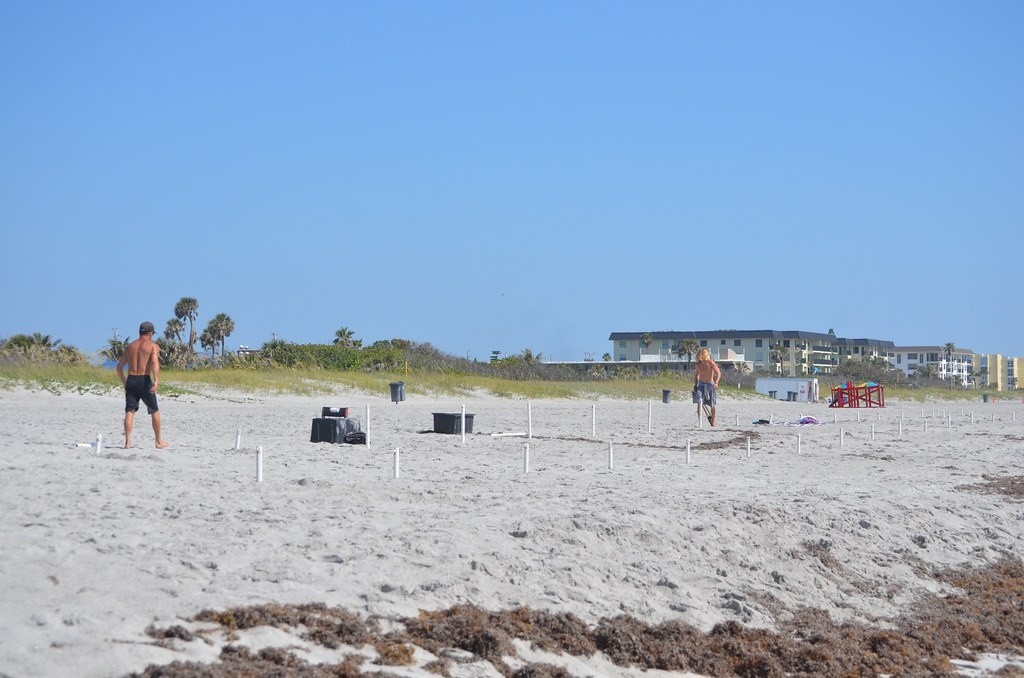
983 395 989 402
793 393 798 402
768 391 777 399
787 391 793 401
662 390 671 403
389 381 405 402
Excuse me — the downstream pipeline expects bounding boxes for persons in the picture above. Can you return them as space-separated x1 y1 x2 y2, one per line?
694 348 721 427
116 321 169 449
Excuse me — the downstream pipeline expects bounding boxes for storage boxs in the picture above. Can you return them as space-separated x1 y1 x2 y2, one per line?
310 418 361 444
431 412 475 434
